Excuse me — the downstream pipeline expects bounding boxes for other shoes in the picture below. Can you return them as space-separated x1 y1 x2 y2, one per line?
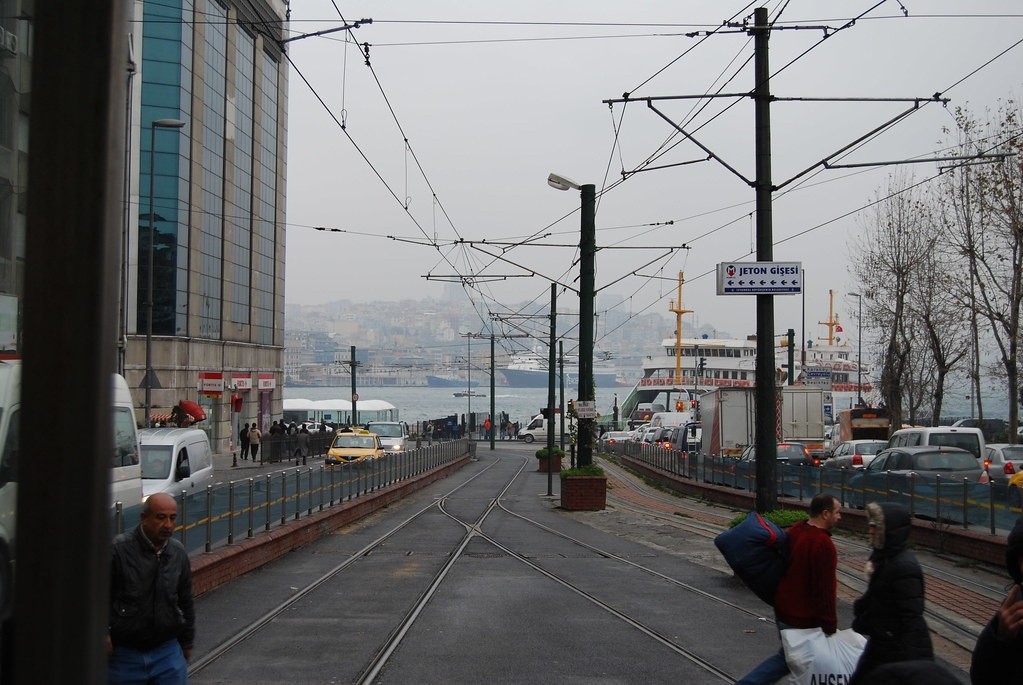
245 459 248 460
241 456 243 459
296 461 298 466
253 460 256 462
303 464 306 465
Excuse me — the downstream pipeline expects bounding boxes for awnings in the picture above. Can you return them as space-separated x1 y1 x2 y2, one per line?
179 400 206 426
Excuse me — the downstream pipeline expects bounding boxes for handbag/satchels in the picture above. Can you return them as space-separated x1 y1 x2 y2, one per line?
714 512 786 605
781 628 867 685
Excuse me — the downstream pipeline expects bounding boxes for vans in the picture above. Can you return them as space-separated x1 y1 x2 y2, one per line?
0 357 143 564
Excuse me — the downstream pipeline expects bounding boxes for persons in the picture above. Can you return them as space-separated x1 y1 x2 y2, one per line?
736 492 842 685
846 502 934 685
969 516 1023 685
240 415 519 466
107 492 194 685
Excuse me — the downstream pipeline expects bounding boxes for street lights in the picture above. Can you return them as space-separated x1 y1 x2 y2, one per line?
547 172 596 468
848 292 862 408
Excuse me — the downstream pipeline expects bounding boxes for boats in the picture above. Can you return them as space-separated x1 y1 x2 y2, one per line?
453 391 486 398
637 269 872 425
498 346 616 388
426 375 479 388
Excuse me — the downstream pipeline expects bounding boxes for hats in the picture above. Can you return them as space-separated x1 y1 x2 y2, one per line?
869 516 875 527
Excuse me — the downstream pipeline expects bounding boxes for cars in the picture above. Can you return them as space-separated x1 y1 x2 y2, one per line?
366 421 410 454
325 427 383 470
986 444 1023 501
137 428 216 507
844 445 991 522
1006 469 1023 507
600 386 1023 473
298 423 333 433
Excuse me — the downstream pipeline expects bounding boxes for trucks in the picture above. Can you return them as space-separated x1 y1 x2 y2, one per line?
517 414 578 444
